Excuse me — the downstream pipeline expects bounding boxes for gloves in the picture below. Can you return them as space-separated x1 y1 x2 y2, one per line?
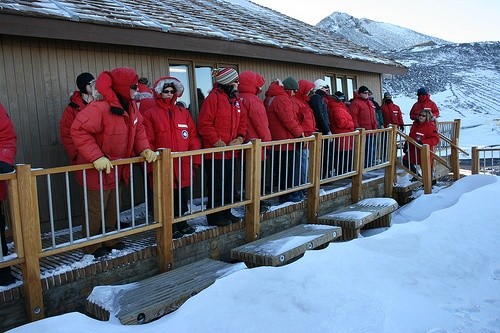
141 149 157 163
400 125 404 131
213 136 243 147
93 157 114 174
403 140 423 153
298 132 306 148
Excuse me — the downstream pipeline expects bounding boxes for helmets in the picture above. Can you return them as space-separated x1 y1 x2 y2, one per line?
358 86 369 93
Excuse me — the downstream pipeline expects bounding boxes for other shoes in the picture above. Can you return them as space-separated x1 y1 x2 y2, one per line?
411 174 421 181
84 241 125 258
207 213 242 226
260 192 304 214
172 228 195 239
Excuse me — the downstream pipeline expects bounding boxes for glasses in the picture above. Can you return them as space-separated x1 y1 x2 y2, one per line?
362 91 369 94
326 90 328 92
130 85 138 90
419 114 427 117
163 90 175 94
386 96 392 98
292 89 297 93
234 79 239 83
342 95 346 98
87 81 95 86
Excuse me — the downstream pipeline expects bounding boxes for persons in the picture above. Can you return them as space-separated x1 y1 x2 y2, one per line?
328 91 354 179
197 67 247 227
141 77 202 239
263 77 332 202
0 103 17 286
61 72 153 233
348 85 384 167
237 71 272 214
402 88 440 185
380 92 405 132
60 69 158 256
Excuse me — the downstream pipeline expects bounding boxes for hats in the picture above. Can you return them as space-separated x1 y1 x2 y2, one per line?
210 67 238 85
384 92 390 98
110 67 139 102
417 88 427 95
282 77 327 90
76 72 95 94
333 91 344 98
